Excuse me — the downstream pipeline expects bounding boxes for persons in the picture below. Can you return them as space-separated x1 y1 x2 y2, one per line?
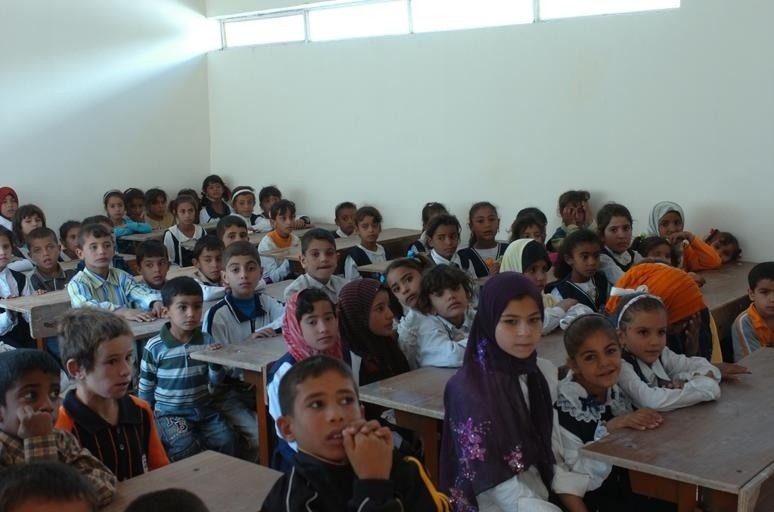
1 188 207 509
440 190 773 509
137 177 476 471
260 355 455 509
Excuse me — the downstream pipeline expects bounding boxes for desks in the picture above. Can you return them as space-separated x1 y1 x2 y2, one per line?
0 217 774 512
